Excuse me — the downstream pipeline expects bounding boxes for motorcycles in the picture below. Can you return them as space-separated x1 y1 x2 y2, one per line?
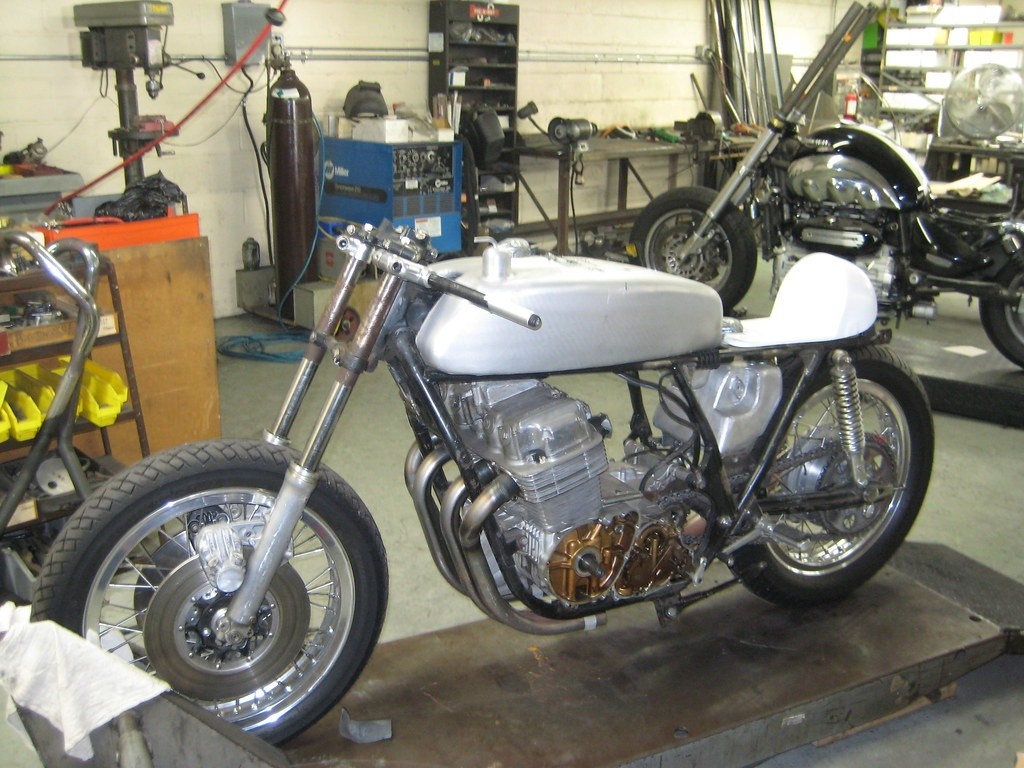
627 0 1024 368
30 217 935 747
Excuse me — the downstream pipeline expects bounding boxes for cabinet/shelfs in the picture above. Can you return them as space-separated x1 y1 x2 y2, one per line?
874 0 1024 151
0 258 168 581
428 0 520 234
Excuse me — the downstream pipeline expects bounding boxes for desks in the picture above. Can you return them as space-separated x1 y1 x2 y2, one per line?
514 133 756 256
925 137 1023 182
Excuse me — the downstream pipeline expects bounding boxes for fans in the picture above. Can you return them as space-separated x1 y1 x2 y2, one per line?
946 64 1024 146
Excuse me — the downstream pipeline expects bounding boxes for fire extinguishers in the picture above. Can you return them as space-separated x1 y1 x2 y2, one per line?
843 84 859 121
